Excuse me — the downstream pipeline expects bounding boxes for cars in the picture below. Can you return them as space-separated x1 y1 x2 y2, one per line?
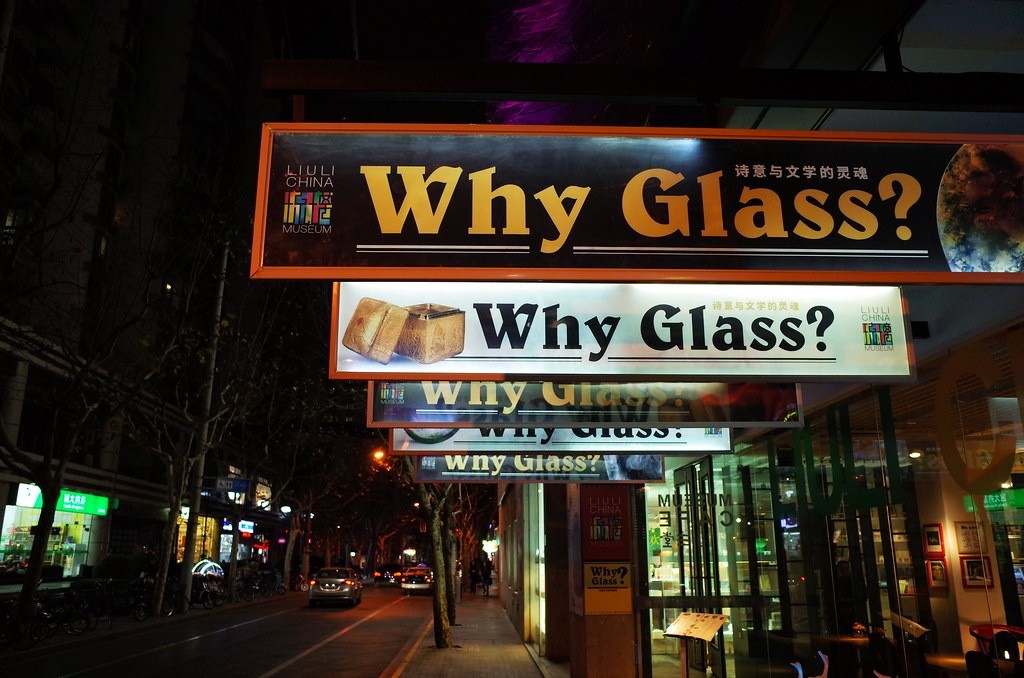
402 563 436 595
374 563 402 589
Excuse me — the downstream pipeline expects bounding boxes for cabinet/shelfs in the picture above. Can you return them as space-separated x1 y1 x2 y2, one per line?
856 515 918 639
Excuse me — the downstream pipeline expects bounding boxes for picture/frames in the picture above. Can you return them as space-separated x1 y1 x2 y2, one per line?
922 523 945 557
926 557 949 590
954 519 989 555
960 556 995 589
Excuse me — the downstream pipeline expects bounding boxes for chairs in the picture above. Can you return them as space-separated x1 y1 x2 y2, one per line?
969 623 1024 678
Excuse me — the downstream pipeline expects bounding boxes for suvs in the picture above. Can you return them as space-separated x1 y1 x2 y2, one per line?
309 568 362 610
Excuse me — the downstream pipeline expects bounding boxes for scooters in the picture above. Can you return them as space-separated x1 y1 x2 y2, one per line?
191 569 287 610
0 570 152 650
291 565 309 592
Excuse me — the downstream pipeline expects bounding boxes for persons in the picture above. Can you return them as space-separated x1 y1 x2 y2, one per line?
835 559 852 634
469 560 479 593
93 543 178 615
196 549 261 600
479 554 493 597
